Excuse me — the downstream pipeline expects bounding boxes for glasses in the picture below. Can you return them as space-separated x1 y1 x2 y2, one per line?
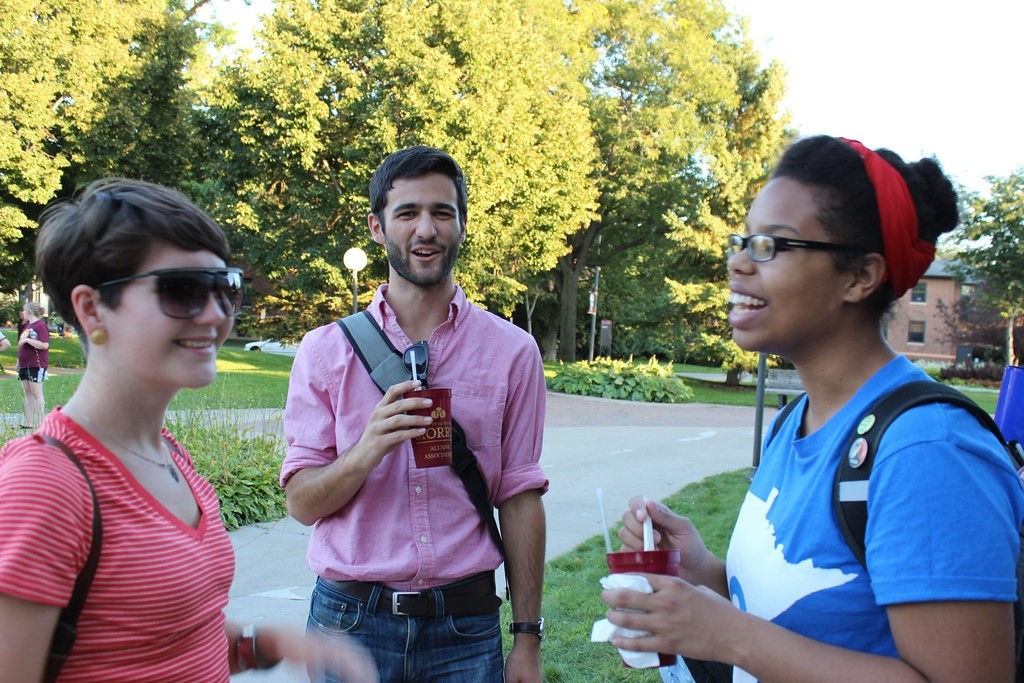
402 341 429 391
727 234 866 262
91 267 246 320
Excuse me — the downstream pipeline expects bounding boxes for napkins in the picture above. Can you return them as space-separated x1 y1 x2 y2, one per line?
590 573 660 669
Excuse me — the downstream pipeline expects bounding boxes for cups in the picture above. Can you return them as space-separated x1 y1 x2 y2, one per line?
402 387 453 468
606 548 677 668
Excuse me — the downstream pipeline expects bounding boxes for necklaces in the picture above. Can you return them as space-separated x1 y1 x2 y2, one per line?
70 400 179 483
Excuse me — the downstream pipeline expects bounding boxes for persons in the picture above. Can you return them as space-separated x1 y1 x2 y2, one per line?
1 180 379 683
601 136 1024 683
280 147 550 682
0 330 12 352
16 301 50 429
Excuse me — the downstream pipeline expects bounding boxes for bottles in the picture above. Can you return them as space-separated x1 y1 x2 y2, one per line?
30 329 38 340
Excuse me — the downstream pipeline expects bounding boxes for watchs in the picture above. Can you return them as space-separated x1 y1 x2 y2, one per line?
508 618 545 640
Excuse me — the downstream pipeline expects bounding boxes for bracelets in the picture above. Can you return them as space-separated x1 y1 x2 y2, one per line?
233 621 282 671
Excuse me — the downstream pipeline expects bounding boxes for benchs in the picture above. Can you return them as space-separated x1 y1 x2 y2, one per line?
763 369 807 410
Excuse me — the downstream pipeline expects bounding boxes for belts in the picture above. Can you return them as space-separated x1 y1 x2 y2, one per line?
324 568 504 617
